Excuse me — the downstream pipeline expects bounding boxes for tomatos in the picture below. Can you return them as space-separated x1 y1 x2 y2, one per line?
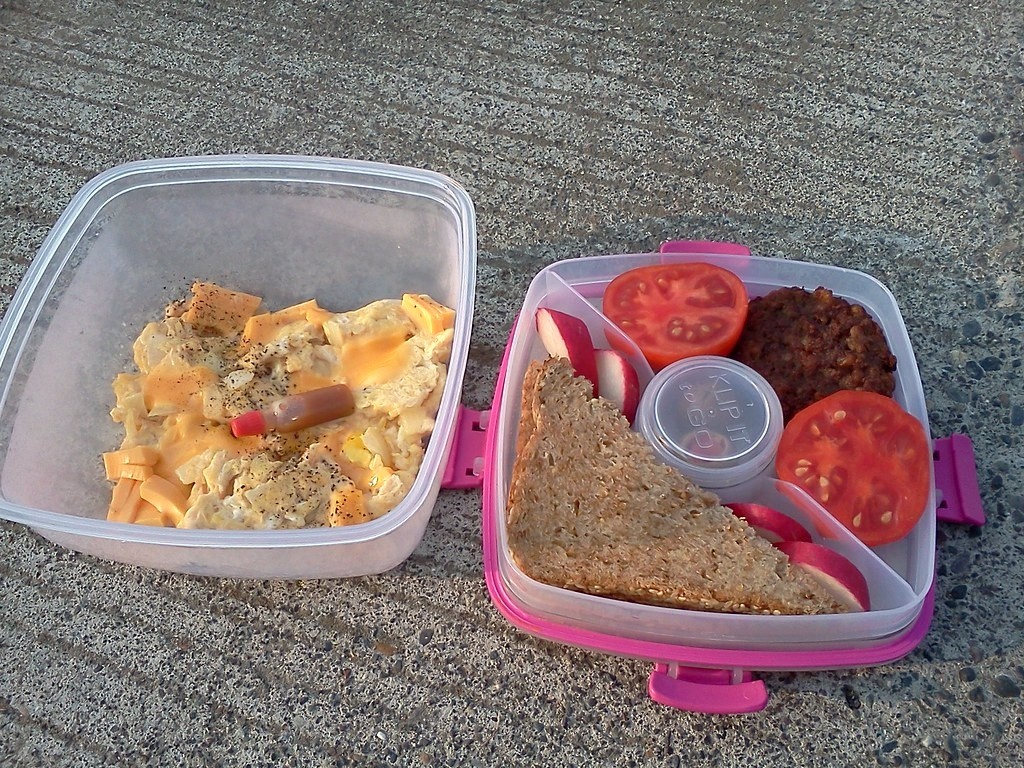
776 388 932 546
602 262 749 370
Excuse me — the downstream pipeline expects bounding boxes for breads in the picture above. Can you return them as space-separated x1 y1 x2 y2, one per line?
504 355 849 615
